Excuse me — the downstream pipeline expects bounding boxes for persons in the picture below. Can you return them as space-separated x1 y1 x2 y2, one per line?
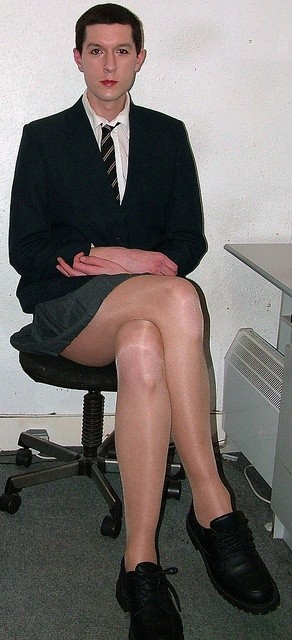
6 3 281 640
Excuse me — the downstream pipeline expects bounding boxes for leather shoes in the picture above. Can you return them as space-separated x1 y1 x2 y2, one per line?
186 498 280 615
116 555 184 640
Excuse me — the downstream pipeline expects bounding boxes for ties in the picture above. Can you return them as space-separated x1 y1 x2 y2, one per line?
101 122 122 207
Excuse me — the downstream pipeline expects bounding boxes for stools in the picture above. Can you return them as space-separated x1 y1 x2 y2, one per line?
222 243 292 552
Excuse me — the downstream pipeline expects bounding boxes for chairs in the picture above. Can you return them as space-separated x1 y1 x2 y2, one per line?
0 348 186 538
